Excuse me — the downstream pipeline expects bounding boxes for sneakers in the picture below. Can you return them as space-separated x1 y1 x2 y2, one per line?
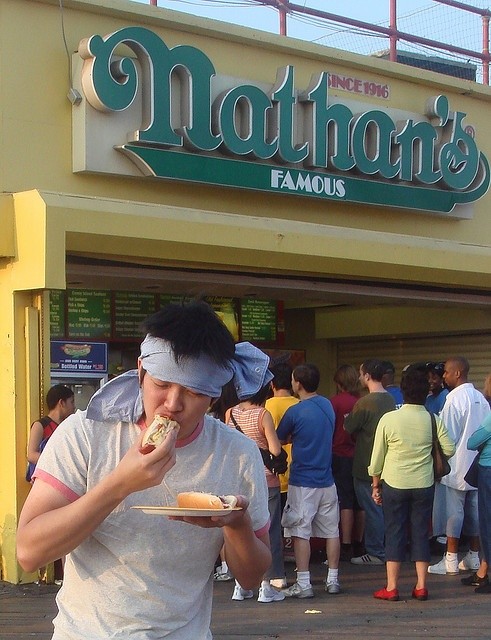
258 588 285 602
412 585 428 601
461 574 489 586
428 556 459 576
374 589 399 601
475 583 491 593
270 579 287 588
280 583 314 598
459 551 480 570
351 554 385 565
232 586 253 601
213 565 234 581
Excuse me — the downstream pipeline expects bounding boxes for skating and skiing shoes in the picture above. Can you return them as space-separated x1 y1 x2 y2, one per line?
325 582 340 594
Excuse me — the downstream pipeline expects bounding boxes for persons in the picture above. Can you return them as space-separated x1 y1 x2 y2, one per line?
424 359 490 573
208 383 240 584
226 377 285 604
466 371 491 595
26 385 76 589
345 359 397 568
260 362 301 589
372 359 402 406
367 364 456 602
420 361 453 413
278 363 340 599
15 310 272 640
326 365 367 563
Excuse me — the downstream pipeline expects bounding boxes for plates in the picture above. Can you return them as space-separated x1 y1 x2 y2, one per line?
129 505 244 517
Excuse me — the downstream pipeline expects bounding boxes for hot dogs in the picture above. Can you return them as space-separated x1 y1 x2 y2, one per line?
140 416 181 453
177 491 237 510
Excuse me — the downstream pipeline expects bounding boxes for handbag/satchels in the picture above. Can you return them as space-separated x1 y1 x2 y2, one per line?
427 410 451 478
464 452 481 487
259 448 288 477
